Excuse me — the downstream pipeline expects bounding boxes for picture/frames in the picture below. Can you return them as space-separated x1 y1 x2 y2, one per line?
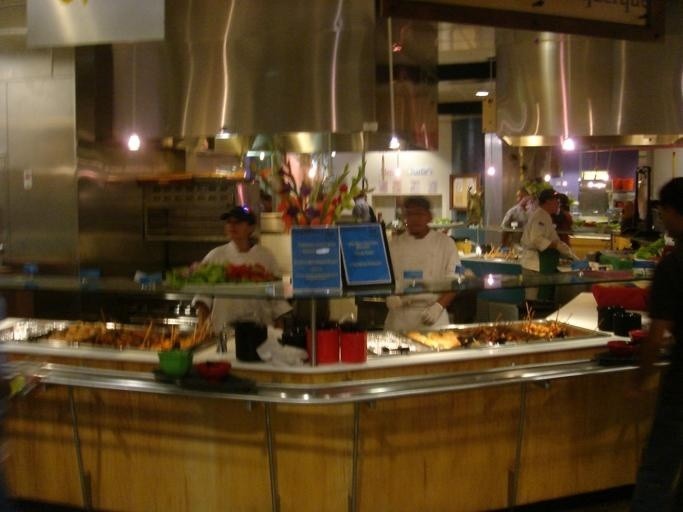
446 171 479 211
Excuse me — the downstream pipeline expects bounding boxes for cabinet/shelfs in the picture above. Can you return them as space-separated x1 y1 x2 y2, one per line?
567 231 616 260
0 273 683 512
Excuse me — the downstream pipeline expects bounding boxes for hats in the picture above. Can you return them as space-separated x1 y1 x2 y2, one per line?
220 206 256 225
538 189 565 204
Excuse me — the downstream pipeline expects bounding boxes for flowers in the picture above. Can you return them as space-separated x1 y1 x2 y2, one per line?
253 160 376 228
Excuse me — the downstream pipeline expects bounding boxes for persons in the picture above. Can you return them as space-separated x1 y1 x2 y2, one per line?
189 205 292 341
385 196 465 332
553 193 575 248
519 188 582 305
499 187 535 245
626 175 682 512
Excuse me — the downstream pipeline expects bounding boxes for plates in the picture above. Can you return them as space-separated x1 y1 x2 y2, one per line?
364 328 437 358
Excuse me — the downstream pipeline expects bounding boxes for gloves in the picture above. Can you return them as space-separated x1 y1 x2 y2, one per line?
421 303 444 326
